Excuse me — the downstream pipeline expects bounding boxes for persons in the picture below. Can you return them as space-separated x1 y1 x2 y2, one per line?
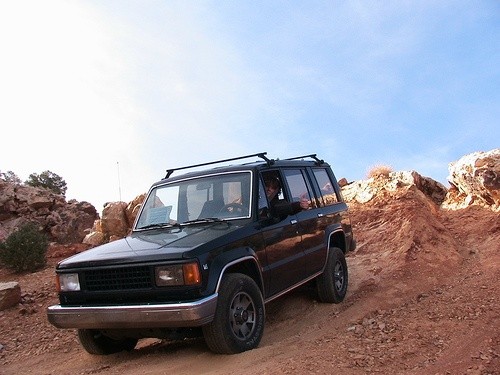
228 174 309 217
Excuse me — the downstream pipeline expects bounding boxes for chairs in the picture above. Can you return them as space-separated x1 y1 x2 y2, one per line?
197 200 230 219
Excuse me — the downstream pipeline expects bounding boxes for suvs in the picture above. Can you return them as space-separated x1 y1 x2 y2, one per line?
47 151 356 353
